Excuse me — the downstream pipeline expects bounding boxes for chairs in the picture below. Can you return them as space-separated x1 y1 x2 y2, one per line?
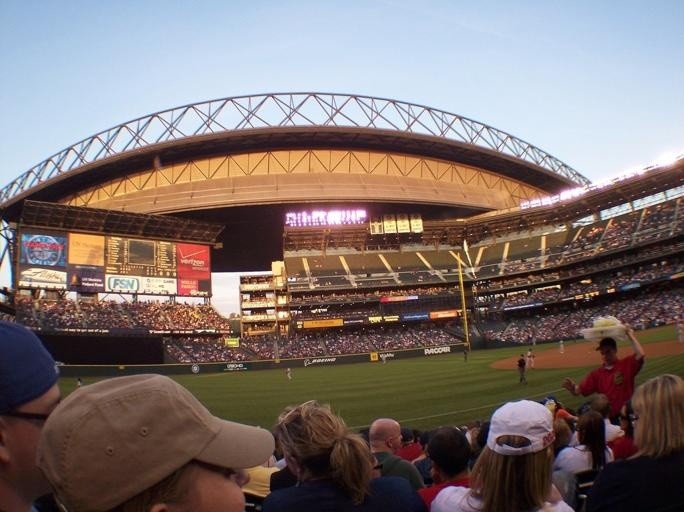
569 470 597 512
582 451 684 511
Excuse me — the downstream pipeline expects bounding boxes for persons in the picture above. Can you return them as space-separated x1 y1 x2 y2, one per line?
1 194 684 364
561 323 646 418
518 354 528 385
34 374 276 512
286 367 292 380
527 349 535 368
0 321 65 512
261 375 683 512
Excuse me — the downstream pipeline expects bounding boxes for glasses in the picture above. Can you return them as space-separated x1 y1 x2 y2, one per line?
616 413 639 421
575 425 584 432
279 398 318 455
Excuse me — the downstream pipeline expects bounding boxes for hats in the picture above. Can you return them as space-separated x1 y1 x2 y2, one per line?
486 399 558 456
34 373 277 511
595 337 616 351
0 319 61 412
